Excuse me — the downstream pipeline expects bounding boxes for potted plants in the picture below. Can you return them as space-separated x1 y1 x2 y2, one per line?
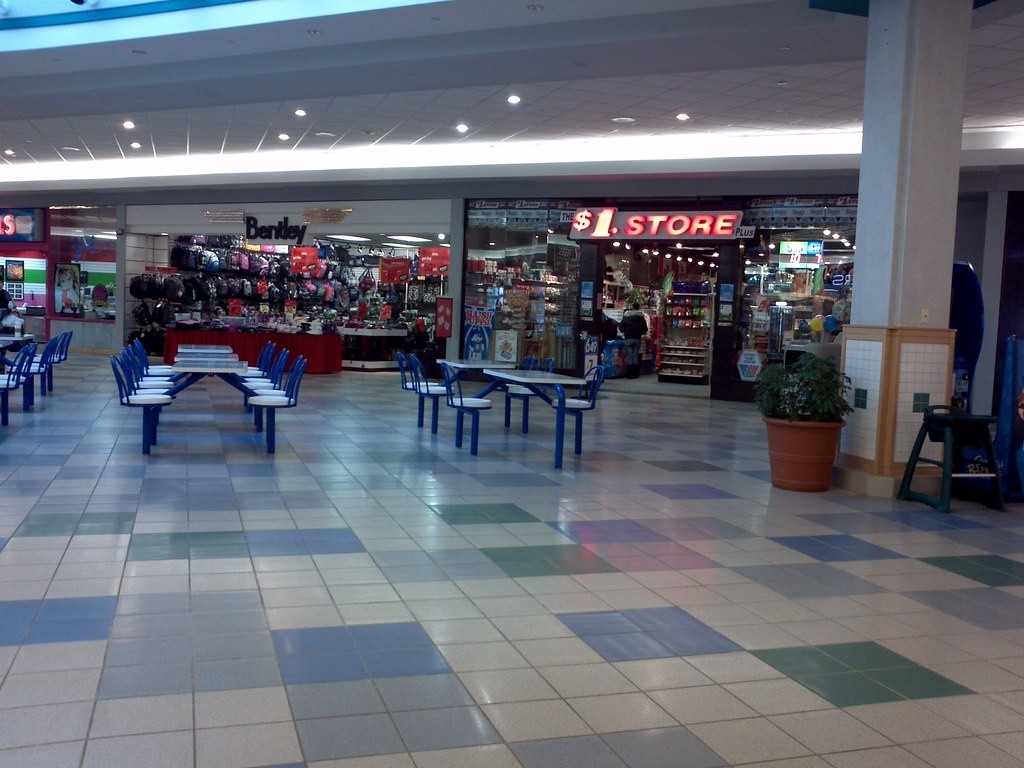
753 352 856 494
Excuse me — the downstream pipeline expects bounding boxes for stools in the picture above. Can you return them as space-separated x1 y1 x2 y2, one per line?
897 404 1008 515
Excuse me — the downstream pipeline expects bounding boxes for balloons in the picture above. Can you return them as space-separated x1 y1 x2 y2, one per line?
796 297 851 340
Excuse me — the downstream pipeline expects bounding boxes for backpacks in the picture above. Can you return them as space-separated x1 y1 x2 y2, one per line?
127 236 448 354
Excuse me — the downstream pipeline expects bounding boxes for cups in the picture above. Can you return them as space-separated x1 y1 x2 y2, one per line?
466 260 497 271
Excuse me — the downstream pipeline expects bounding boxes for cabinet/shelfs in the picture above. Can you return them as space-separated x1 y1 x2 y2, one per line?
82 294 116 315
604 279 834 384
404 273 577 363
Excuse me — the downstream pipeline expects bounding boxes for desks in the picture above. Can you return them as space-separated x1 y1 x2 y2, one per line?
337 327 408 371
161 360 259 399
0 333 35 341
168 353 249 383
178 344 234 354
0 340 14 375
484 368 588 471
436 358 516 448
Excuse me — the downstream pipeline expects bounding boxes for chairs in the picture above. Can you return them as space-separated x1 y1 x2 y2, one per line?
110 355 172 457
249 354 304 432
248 358 308 455
0 329 73 427
551 365 606 456
244 340 272 407
122 349 177 426
133 337 172 369
507 357 556 434
247 343 277 414
439 362 492 457
246 348 287 415
133 340 174 377
128 345 176 412
248 350 290 425
118 351 177 446
494 356 537 428
396 351 440 427
409 353 456 435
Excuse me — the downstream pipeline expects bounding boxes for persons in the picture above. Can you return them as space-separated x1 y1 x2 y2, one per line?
618 302 648 379
0 275 19 374
599 310 610 383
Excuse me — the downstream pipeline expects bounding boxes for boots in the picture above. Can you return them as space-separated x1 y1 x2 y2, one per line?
634 364 640 379
623 365 634 378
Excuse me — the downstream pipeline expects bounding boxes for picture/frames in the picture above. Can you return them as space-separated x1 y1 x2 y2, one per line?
6 260 24 281
